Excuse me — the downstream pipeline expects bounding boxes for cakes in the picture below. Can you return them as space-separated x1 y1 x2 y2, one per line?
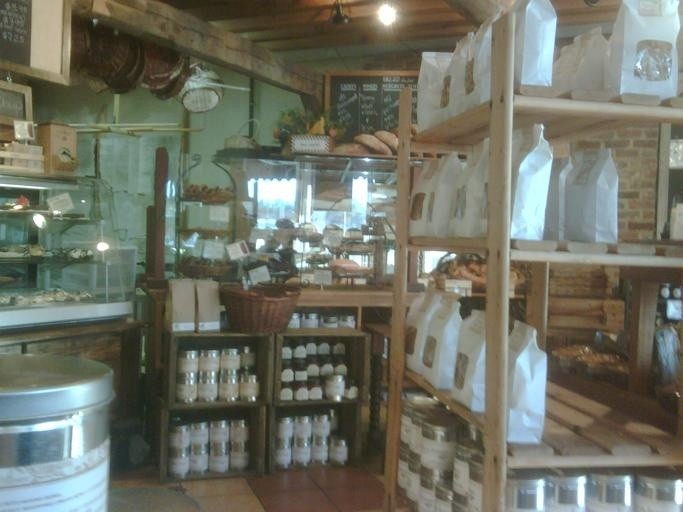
253 214 363 273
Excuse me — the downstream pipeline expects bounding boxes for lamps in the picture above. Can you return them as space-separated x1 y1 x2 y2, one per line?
327 0 352 28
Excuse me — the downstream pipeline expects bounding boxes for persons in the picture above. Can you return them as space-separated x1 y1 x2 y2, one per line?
257 218 298 278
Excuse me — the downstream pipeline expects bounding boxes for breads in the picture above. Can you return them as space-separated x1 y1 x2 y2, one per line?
560 344 628 374
439 262 519 284
333 122 418 157
183 183 234 203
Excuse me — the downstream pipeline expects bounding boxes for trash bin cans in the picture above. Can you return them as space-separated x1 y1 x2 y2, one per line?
0 352 117 512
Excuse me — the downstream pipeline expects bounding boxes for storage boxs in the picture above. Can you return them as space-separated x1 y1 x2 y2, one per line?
36 122 78 175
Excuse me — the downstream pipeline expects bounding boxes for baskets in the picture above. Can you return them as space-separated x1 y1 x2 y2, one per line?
218 283 301 333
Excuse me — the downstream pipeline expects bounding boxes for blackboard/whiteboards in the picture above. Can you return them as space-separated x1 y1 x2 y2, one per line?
0 0 32 68
323 70 420 146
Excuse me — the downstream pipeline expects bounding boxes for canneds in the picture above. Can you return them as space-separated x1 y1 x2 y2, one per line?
288 312 355 330
635 471 683 512
397 397 485 512
177 345 260 400
504 472 547 511
168 415 250 475
280 342 359 401
547 475 588 512
588 473 635 512
275 409 349 466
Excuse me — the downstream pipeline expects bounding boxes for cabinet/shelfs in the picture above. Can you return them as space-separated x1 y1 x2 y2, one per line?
271 331 373 474
157 331 275 485
385 11 682 512
0 143 139 305
167 152 424 330
